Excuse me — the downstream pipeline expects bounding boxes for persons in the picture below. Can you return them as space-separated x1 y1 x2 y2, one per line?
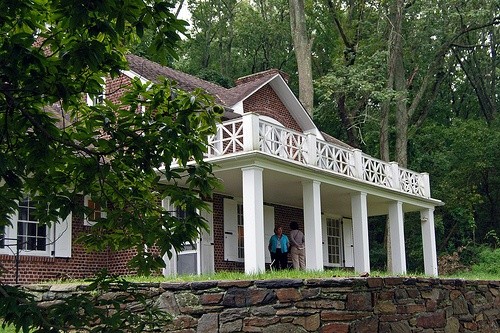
268 225 289 270
288 220 306 270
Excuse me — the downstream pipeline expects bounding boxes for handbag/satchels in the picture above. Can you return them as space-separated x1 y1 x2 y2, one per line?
288 244 293 254
